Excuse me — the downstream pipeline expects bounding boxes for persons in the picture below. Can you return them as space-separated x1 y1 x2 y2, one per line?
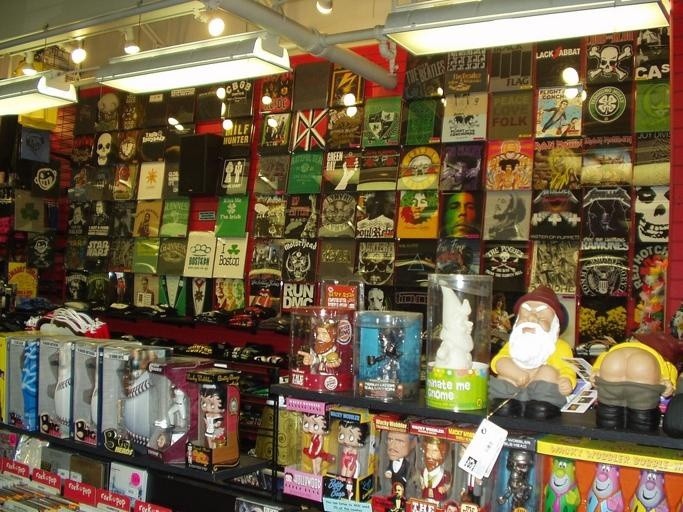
288 247 307 280
318 191 526 241
297 315 343 376
337 419 370 478
301 413 683 512
437 238 477 275
420 436 453 504
497 159 520 191
543 456 670 511
369 322 405 380
89 201 112 226
384 431 417 497
540 100 578 137
214 278 245 312
303 413 336 474
140 213 151 236
32 240 50 266
250 237 280 271
459 470 483 506
497 450 535 510
168 381 226 449
589 329 683 433
135 276 154 306
489 286 578 422
68 205 89 235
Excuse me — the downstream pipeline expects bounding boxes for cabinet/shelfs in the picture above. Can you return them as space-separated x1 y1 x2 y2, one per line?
92 295 307 450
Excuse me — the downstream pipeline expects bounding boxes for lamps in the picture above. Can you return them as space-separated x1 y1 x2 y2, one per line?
96 33 293 99
384 0 669 60
14 8 231 77
0 66 80 128
316 1 336 15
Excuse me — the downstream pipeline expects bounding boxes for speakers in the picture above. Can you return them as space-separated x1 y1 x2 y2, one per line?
178 134 224 197
